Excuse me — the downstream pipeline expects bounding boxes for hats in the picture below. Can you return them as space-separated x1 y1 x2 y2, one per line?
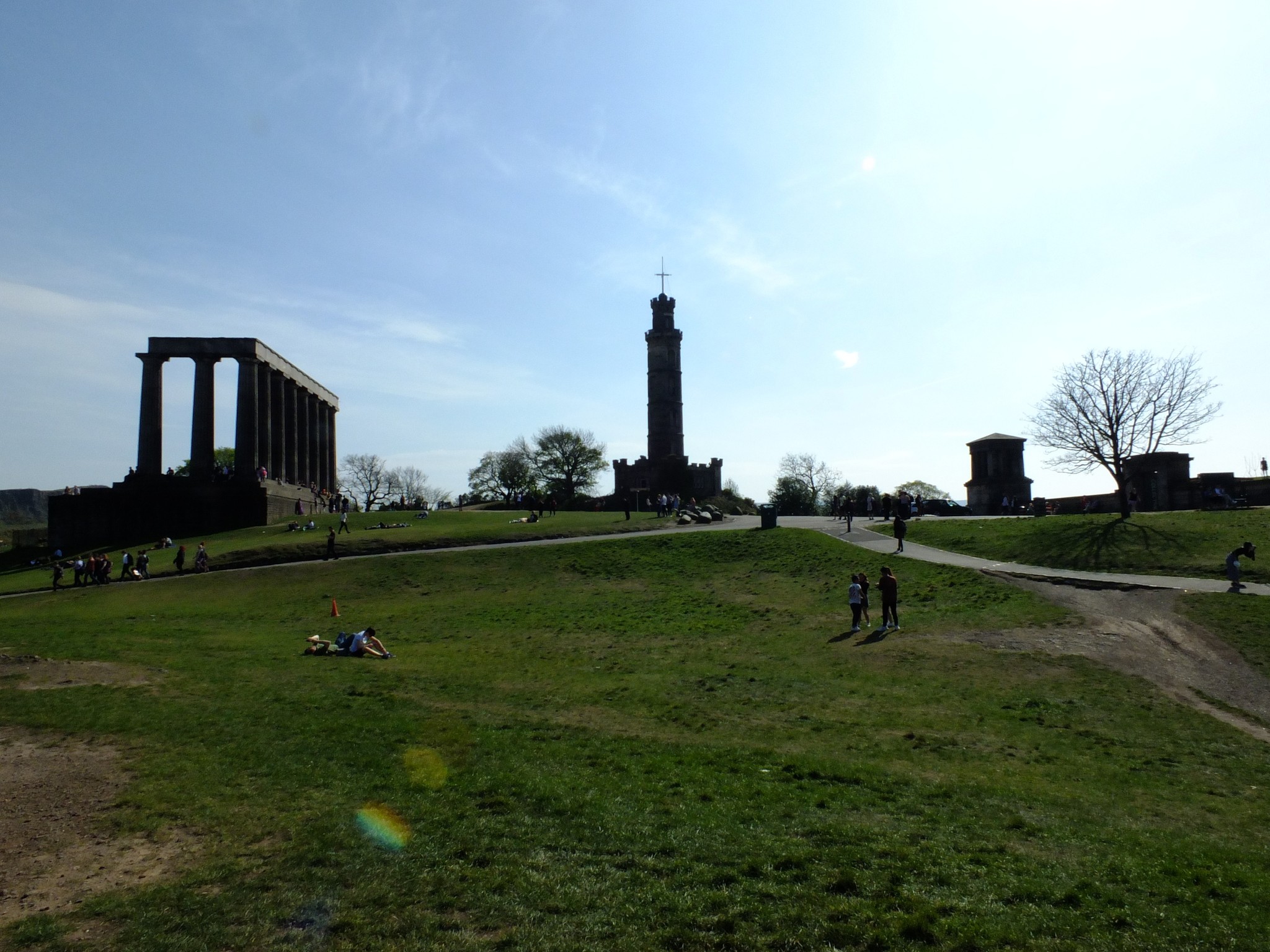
305 647 310 655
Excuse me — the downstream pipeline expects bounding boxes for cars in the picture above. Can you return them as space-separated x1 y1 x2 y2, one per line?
912 498 973 516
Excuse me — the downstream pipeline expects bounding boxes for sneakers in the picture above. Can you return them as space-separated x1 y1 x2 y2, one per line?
851 625 861 631
857 621 861 626
387 652 396 658
866 622 871 627
381 654 389 659
886 622 894 627
877 626 889 631
894 626 901 631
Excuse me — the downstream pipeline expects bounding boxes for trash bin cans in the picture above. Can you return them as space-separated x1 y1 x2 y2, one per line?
1033 497 1046 517
760 503 777 529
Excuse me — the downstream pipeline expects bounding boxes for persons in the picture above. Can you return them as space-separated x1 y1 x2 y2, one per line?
877 567 895 627
349 627 397 659
855 572 870 629
874 566 901 631
848 574 865 631
304 631 356 657
16 453 1270 596
